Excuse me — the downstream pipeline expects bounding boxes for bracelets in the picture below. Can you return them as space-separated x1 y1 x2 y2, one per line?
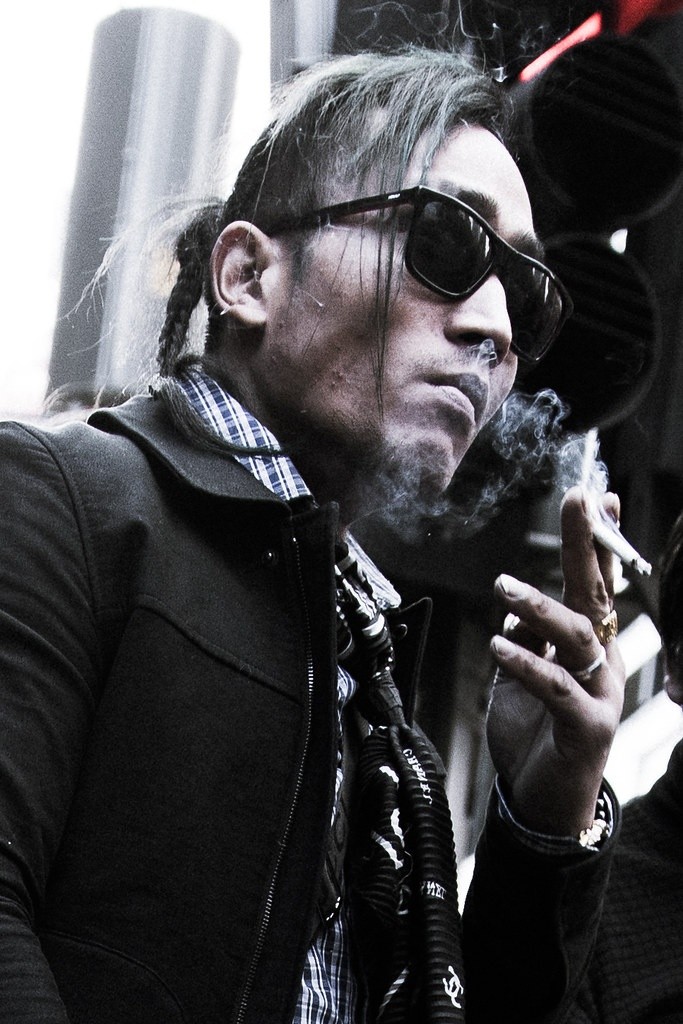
498 779 611 853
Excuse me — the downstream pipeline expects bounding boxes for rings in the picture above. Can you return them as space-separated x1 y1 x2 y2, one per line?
555 641 606 684
590 609 619 643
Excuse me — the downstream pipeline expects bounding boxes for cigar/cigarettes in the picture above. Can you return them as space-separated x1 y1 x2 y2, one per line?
589 506 652 577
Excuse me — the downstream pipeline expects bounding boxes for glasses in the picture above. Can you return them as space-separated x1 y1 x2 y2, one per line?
263 184 573 364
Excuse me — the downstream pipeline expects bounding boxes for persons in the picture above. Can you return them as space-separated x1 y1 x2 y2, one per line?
1 47 682 1023
